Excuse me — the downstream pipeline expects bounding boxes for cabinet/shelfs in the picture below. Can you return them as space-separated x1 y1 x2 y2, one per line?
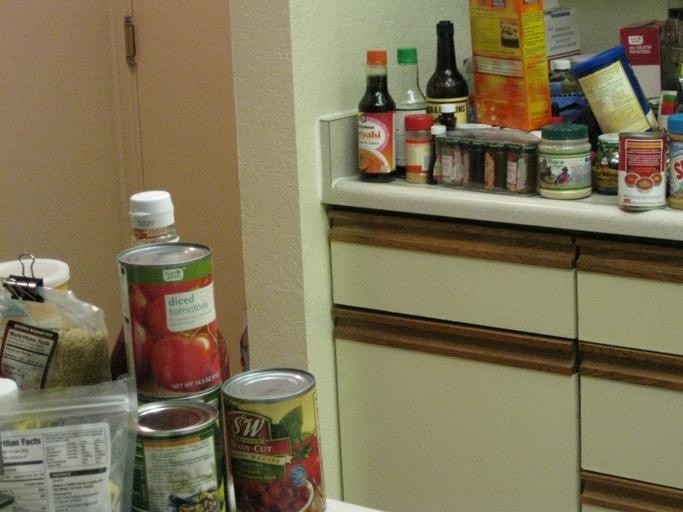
326 208 683 511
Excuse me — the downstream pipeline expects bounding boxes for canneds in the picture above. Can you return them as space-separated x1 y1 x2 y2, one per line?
116 239 224 400
121 401 231 511
617 132 668 212
220 367 327 511
439 137 537 194
136 386 230 489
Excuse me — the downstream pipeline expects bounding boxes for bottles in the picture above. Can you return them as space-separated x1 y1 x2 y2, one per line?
357 47 456 185
125 189 181 246
425 20 469 124
549 59 586 120
